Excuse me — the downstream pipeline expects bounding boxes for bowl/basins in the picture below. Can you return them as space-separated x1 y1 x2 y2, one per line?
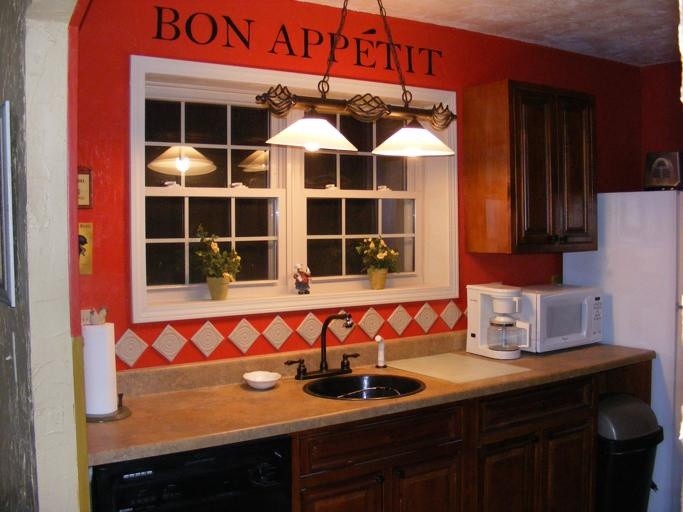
242 370 282 390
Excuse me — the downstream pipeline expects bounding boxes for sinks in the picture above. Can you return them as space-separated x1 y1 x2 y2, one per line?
304 375 426 400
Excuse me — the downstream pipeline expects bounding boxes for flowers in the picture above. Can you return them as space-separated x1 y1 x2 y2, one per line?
355 233 399 273
194 223 242 282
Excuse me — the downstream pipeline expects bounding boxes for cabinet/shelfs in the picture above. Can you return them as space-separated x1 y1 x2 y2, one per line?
292 398 473 512
462 77 599 256
473 373 597 512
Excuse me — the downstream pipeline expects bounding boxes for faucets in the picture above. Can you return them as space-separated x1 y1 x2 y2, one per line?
320 314 354 372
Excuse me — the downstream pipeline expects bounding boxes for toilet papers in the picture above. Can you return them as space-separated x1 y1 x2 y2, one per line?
81 322 118 415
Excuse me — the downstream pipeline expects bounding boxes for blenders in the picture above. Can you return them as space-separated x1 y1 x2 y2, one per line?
466 281 531 360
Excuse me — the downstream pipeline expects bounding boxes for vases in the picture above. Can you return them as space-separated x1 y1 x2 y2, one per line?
206 277 230 300
367 268 388 290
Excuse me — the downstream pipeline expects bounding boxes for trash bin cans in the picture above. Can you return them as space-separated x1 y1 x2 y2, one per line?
596 393 664 511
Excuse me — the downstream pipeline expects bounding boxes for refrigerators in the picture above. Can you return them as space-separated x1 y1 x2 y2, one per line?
562 190 683 511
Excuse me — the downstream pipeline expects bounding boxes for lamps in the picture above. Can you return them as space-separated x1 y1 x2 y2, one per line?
147 146 218 176
264 114 359 155
238 150 270 173
370 120 456 159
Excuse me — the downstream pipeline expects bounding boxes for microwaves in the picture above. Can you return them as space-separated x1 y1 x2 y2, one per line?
510 283 604 351
